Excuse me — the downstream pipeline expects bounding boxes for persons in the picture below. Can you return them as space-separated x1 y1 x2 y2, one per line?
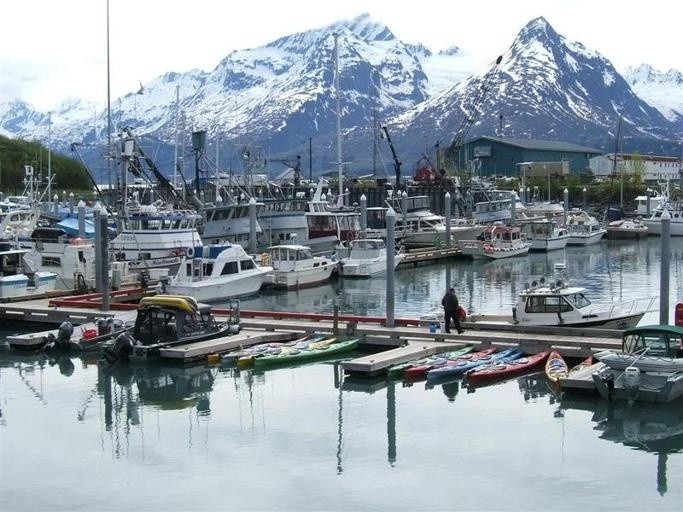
457 305 466 322
442 287 465 334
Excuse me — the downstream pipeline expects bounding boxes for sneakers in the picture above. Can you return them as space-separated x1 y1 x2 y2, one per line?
458 330 464 334
446 331 451 333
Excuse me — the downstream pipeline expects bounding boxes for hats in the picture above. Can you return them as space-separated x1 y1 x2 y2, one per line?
449 288 455 291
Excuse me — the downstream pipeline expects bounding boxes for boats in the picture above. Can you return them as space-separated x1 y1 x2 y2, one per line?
69 294 240 362
386 345 552 389
644 201 683 236
509 208 608 258
545 350 568 383
567 354 593 375
607 218 649 239
591 325 683 402
207 335 361 367
419 275 660 330
